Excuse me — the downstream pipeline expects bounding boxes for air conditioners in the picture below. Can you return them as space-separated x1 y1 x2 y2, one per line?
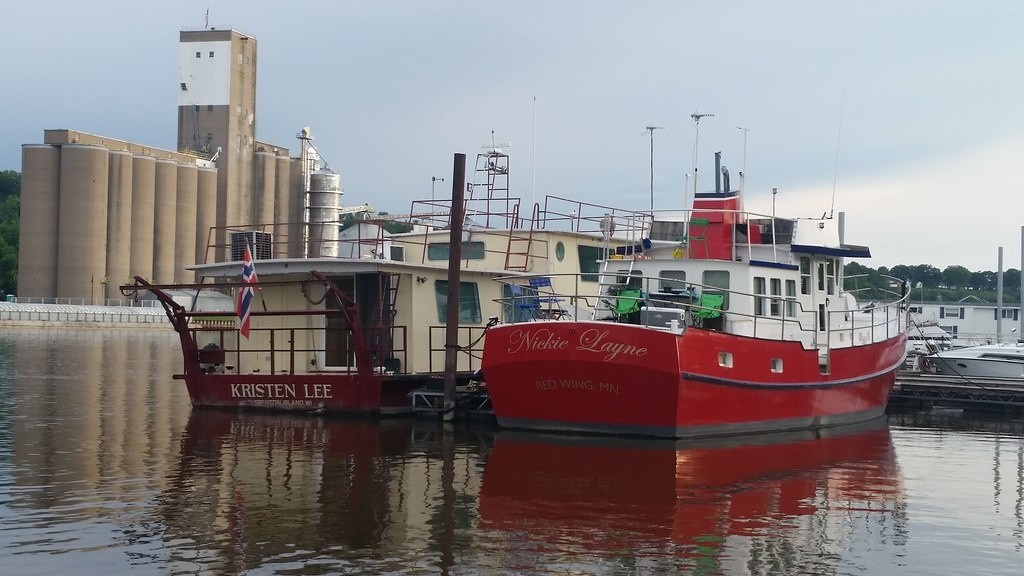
229 231 274 261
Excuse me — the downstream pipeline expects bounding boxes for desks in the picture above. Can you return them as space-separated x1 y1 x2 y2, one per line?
649 292 698 327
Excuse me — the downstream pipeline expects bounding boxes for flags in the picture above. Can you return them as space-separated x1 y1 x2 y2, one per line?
237 244 258 340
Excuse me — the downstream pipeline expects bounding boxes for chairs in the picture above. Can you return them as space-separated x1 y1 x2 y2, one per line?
602 289 641 324
529 277 566 322
692 294 724 331
510 284 536 323
675 217 709 259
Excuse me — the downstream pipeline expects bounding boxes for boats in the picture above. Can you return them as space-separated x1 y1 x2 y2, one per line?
879 226 1024 407
117 147 692 424
482 113 913 441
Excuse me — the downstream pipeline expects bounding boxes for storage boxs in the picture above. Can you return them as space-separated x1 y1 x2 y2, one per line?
641 307 685 328
